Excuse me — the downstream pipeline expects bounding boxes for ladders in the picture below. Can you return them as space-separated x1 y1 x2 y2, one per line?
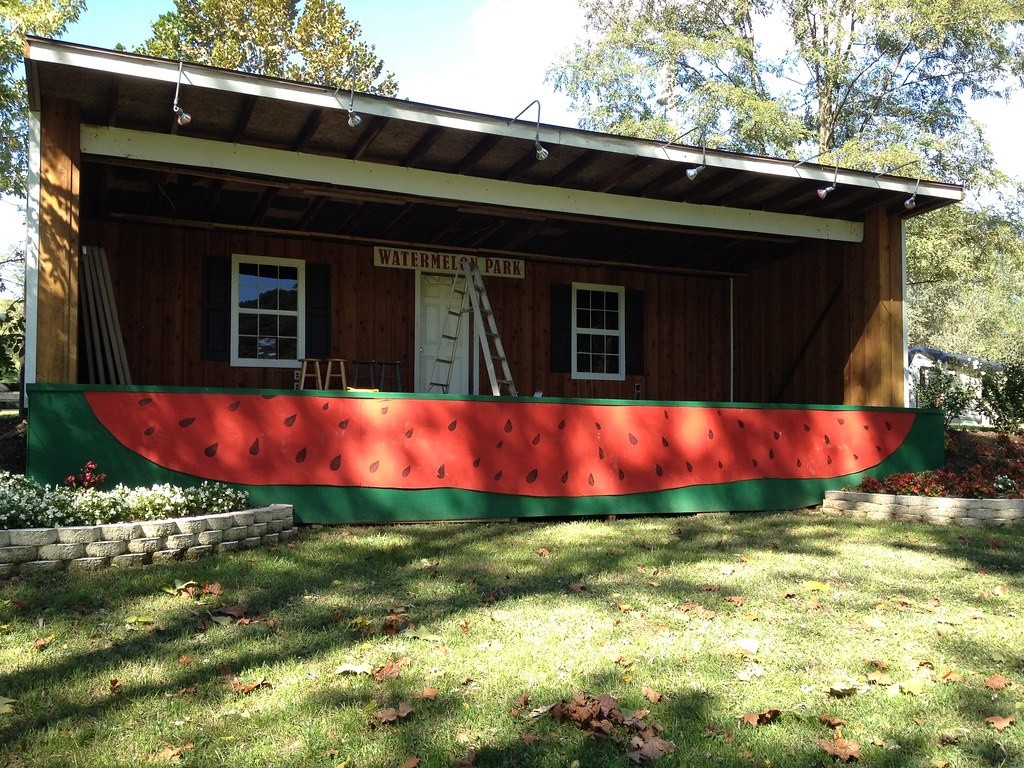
427 261 519 397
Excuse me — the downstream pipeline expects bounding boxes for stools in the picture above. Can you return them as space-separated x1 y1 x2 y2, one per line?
323 359 349 391
379 360 402 392
297 358 323 390
352 359 377 388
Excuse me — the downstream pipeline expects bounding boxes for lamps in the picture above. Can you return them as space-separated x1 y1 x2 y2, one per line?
507 100 549 160
794 148 839 200
173 62 192 125
665 126 706 180
334 64 359 125
875 159 923 209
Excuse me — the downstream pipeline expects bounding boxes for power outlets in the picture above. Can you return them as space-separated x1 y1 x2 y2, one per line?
294 371 300 380
294 383 299 390
